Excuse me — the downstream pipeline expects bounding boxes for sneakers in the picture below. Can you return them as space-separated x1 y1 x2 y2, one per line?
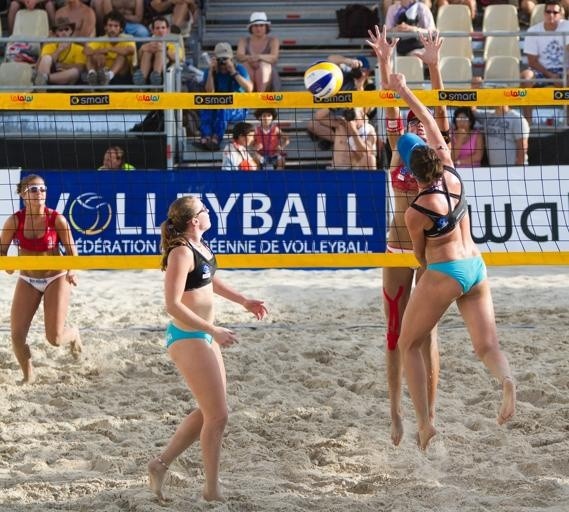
34 72 46 93
151 71 162 93
133 69 145 85
88 72 98 93
96 67 108 90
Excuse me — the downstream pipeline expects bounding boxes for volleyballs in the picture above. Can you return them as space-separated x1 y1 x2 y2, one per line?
303 59 344 99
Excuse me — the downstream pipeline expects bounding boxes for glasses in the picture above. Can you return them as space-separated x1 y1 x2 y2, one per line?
195 205 207 217
23 184 47 193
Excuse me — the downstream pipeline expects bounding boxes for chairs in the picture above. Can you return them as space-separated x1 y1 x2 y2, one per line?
0 0 193 92
381 0 569 91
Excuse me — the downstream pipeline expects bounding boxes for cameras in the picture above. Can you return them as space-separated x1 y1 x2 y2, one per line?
216 57 229 66
344 110 355 121
351 68 361 78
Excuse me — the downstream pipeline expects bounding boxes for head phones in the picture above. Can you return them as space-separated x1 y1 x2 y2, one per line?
104 146 122 161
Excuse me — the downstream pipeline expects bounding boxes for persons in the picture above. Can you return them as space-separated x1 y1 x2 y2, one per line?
145 195 268 502
0 172 82 386
362 23 450 449
1 2 289 169
310 0 569 168
396 70 516 451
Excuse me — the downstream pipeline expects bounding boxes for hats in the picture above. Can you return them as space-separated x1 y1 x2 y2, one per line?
247 11 271 31
213 42 233 58
407 106 431 121
397 132 427 176
49 17 76 38
